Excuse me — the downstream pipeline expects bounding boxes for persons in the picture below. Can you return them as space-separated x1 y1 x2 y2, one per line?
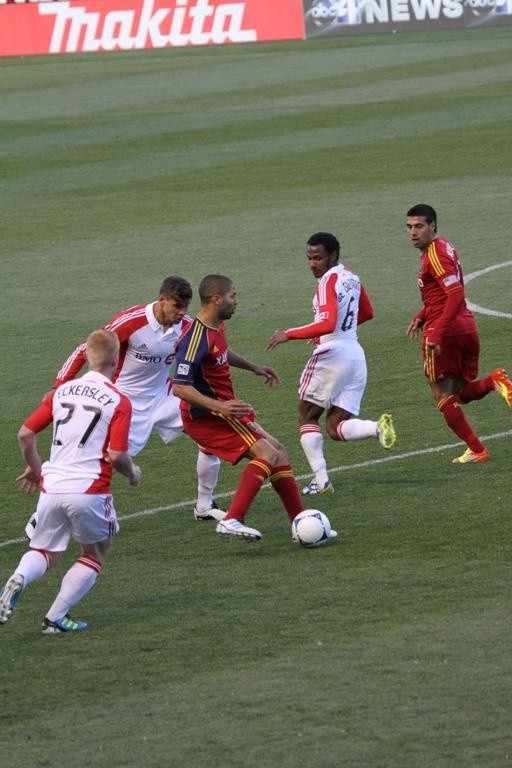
41 276 282 477
0 327 142 635
265 231 396 496
405 203 512 465
172 273 304 540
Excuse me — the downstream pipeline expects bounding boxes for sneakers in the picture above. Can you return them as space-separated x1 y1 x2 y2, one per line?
491 368 512 408
215 518 262 542
376 415 397 449
41 613 88 634
302 478 335 496
452 447 490 463
0 574 24 624
194 501 227 520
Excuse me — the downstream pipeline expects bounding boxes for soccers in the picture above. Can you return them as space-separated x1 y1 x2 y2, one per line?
292 509 331 547
